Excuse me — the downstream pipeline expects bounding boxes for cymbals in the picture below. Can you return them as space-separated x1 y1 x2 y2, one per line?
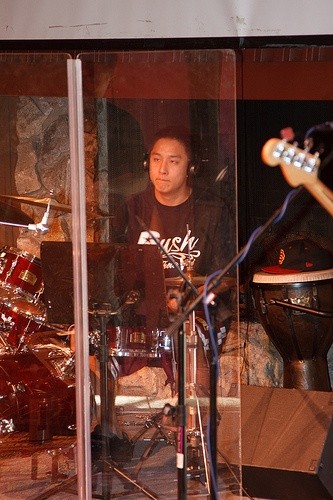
165 275 237 284
0 202 34 224
1 194 106 218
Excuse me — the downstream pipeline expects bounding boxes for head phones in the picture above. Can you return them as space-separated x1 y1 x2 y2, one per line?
143 138 200 176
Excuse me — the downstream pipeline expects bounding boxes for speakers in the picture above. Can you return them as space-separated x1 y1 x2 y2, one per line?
215 383 333 500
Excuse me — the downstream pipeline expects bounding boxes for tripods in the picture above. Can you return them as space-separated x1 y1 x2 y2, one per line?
33 217 213 500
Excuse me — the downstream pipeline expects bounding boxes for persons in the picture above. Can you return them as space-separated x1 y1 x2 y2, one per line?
63 125 237 478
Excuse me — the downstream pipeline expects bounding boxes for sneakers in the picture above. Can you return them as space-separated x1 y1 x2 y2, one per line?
186 445 205 474
62 424 133 462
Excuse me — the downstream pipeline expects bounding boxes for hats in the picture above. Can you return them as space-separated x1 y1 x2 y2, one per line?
261 237 333 274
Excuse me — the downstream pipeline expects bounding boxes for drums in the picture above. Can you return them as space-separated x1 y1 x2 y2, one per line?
1 344 96 437
0 244 46 308
0 297 67 350
253 266 333 391
94 315 165 357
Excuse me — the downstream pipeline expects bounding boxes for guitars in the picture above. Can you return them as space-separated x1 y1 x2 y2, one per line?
260 137 333 219
